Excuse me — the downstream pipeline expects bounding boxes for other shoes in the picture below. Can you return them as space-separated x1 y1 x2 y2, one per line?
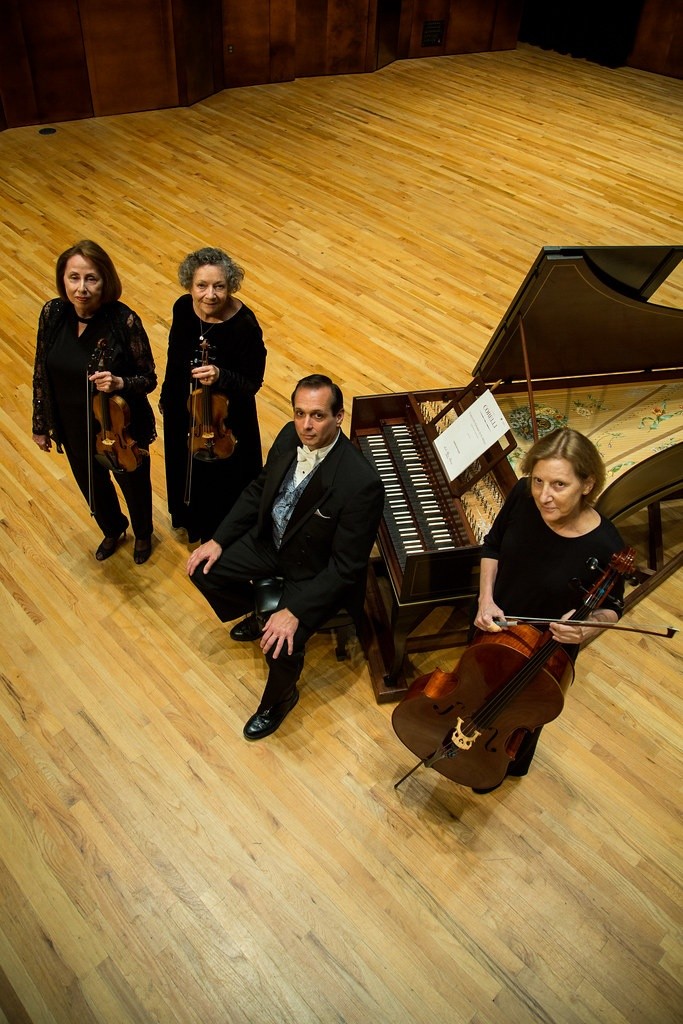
472 776 506 794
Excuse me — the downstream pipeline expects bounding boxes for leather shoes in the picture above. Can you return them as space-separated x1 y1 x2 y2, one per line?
243 686 300 739
230 608 276 641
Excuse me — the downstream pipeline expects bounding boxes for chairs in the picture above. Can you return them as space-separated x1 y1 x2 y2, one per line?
252 570 353 661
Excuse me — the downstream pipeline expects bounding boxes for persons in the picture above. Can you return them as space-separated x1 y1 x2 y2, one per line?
31 241 158 565
186 374 385 739
468 426 626 794
158 247 266 546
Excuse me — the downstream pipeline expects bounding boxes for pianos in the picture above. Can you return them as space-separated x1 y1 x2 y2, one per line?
349 243 683 705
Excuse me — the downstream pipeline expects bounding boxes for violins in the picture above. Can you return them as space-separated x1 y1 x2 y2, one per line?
185 338 237 462
91 339 144 473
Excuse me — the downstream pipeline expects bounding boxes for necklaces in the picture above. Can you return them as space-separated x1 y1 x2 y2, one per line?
200 310 216 340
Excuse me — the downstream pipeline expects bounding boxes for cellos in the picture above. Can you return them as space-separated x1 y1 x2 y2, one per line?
390 546 641 790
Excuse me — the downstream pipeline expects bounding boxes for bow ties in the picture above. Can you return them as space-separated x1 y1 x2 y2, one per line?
296 447 318 467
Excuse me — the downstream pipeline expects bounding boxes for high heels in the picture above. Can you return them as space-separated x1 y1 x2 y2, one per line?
133 536 153 564
95 519 130 561
